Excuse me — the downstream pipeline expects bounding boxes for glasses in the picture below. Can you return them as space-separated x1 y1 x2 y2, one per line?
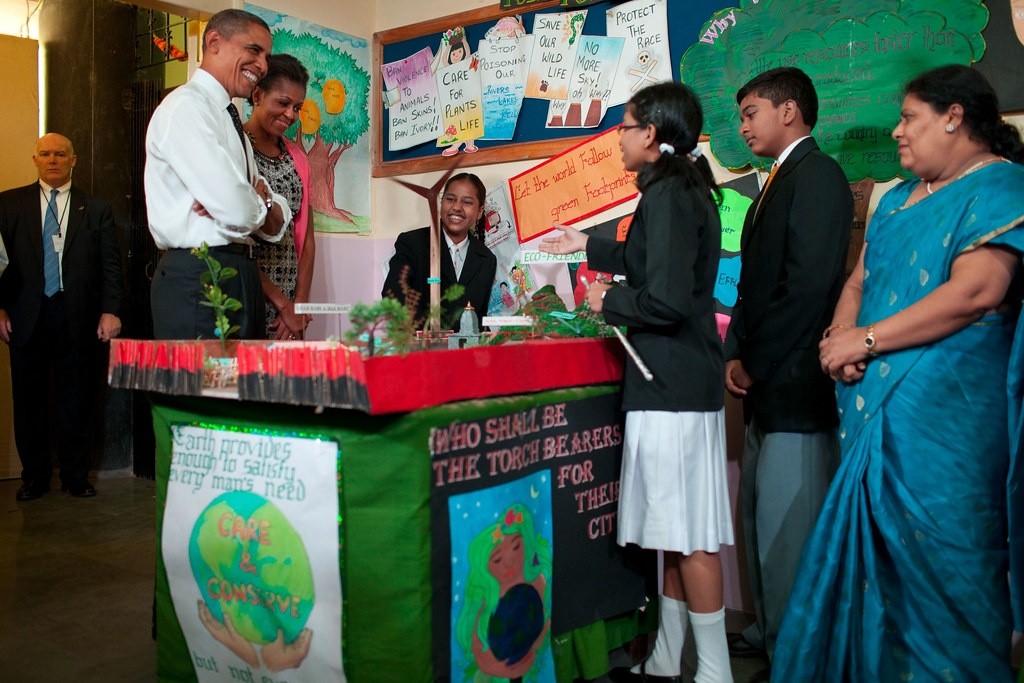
617 124 648 135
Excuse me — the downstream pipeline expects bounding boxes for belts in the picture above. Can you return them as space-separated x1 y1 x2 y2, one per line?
216 242 258 259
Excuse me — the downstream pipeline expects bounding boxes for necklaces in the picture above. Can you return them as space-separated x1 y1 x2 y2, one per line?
926 156 1012 194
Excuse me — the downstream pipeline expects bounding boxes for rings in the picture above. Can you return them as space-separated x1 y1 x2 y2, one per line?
289 335 294 340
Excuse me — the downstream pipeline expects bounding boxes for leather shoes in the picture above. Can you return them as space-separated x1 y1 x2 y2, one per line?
18 478 43 501
62 472 97 497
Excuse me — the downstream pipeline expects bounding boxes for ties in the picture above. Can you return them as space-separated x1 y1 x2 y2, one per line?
44 190 60 298
450 246 458 268
762 165 778 199
227 103 253 184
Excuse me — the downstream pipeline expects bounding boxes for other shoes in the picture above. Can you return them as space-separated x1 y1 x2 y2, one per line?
609 658 682 683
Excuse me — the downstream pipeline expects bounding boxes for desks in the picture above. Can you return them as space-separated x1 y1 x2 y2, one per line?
105 335 660 683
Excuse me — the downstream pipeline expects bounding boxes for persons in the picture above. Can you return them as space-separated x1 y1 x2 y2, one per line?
769 64 1024 683
722 66 855 683
381 173 497 333
242 53 316 340
0 133 124 501
144 6 293 340
537 83 735 683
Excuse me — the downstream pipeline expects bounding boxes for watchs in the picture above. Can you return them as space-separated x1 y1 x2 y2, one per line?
265 192 274 210
864 323 876 357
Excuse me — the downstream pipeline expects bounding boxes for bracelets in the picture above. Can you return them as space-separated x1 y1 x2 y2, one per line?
823 324 845 339
